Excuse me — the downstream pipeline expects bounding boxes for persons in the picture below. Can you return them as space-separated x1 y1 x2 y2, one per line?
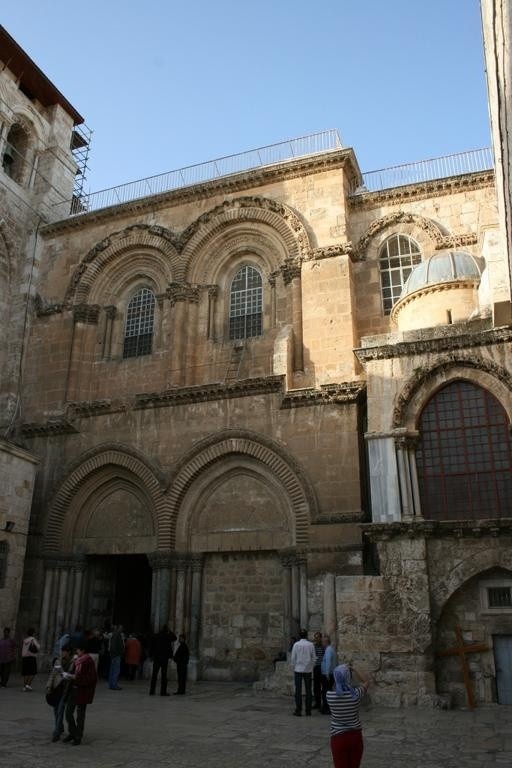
62 644 98 747
47 644 73 743
325 663 369 768
285 628 337 718
1 621 191 696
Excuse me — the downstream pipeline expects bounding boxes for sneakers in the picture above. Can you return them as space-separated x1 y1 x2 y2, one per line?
112 687 121 690
160 692 170 696
306 712 311 715
293 712 301 715
312 704 321 708
52 735 60 741
173 692 183 694
63 734 81 745
150 693 154 695
22 685 32 692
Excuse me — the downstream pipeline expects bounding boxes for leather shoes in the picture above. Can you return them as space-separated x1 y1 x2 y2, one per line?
319 708 330 714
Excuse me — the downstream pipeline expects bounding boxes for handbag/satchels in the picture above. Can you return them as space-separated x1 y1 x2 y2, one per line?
28 639 38 653
46 679 66 706
